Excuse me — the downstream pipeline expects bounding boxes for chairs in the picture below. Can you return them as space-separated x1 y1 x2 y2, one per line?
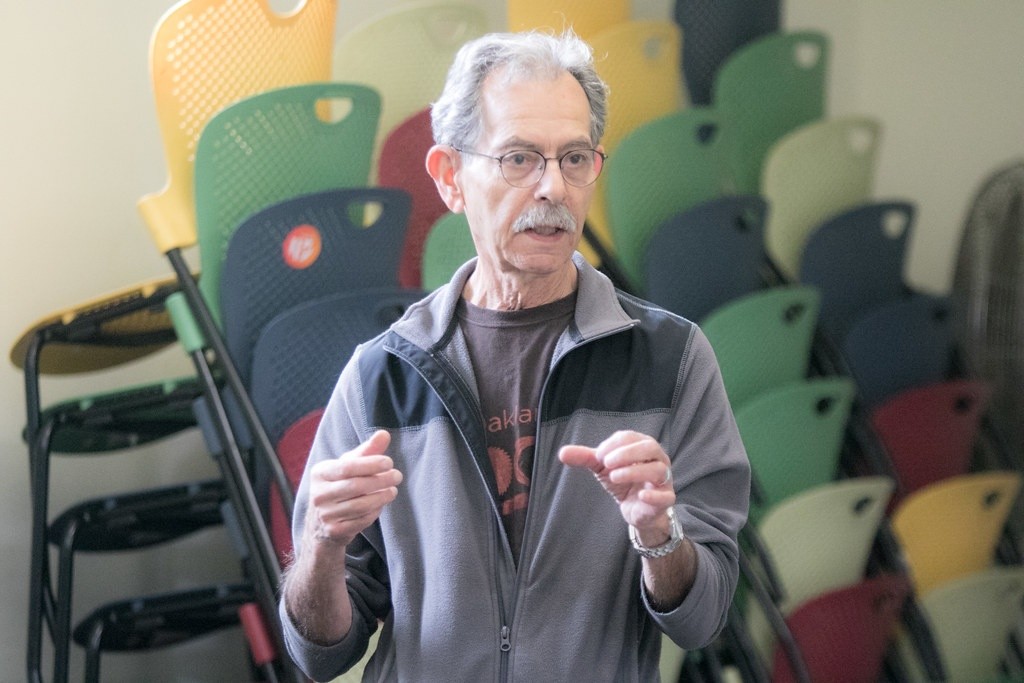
0 0 1024 683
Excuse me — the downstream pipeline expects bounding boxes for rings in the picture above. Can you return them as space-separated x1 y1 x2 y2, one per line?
658 468 672 485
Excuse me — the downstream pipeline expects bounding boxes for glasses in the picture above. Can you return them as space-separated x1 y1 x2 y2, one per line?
451 145 609 188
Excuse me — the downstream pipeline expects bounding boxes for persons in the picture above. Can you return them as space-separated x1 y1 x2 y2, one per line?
278 26 751 683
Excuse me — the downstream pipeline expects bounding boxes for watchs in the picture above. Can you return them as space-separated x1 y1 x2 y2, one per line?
628 505 683 559
